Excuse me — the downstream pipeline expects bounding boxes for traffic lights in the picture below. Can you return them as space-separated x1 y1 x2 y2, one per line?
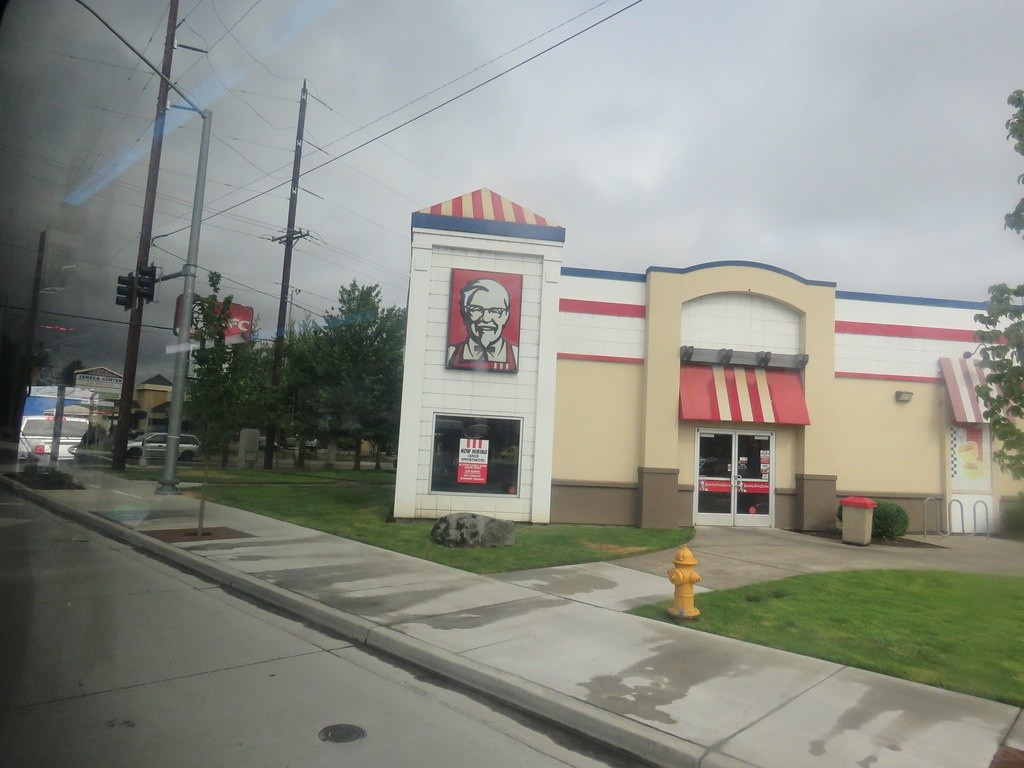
135 263 154 300
115 272 132 307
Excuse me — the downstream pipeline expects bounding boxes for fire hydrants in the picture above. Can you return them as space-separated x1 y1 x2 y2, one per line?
665 544 703 618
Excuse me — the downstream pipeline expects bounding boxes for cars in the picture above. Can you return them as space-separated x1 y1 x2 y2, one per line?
17 414 91 465
127 431 201 462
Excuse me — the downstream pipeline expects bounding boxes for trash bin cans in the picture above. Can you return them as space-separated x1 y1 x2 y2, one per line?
839 496 878 546
